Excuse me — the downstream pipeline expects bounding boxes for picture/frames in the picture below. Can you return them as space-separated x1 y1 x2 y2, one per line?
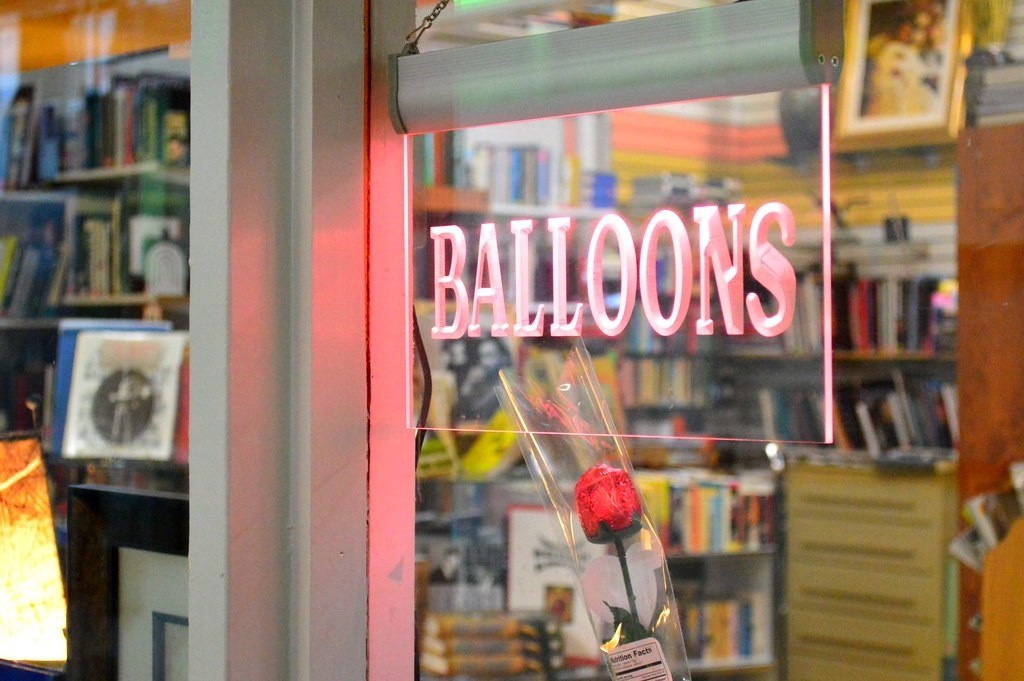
67 487 188 681
833 0 974 156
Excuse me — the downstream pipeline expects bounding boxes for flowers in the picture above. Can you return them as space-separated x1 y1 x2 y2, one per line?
571 461 663 644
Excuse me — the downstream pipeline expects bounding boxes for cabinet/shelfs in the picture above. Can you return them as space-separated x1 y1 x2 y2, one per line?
425 194 959 681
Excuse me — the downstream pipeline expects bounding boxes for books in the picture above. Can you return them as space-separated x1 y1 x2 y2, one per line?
622 304 708 404
1 72 190 319
421 616 563 676
758 370 959 454
637 470 777 552
674 590 757 660
782 270 938 354
